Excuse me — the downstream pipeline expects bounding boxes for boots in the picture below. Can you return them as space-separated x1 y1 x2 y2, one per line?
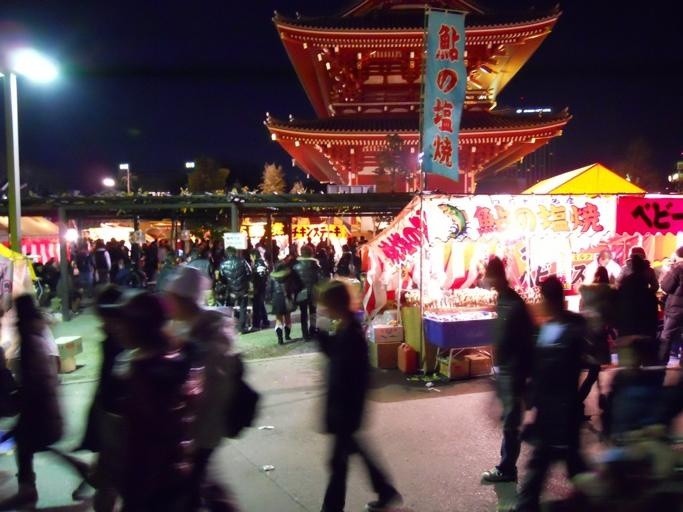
273 327 285 345
283 325 292 341
0 472 40 512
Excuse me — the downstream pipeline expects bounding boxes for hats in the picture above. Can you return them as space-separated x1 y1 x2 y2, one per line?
99 285 169 327
157 265 206 300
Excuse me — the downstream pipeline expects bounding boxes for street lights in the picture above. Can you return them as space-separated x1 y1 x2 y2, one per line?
119 164 130 196
1 44 57 256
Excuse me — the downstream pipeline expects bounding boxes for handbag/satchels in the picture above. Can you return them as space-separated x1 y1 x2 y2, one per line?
294 286 311 304
282 295 299 312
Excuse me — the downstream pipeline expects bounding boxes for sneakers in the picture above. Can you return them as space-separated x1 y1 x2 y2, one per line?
482 466 520 484
364 484 405 512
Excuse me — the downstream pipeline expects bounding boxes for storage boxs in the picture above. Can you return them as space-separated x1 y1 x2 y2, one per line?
369 324 404 342
440 352 469 378
368 342 400 369
464 351 491 377
55 336 83 360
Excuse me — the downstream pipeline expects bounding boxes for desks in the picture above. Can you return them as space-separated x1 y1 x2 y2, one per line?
423 310 499 378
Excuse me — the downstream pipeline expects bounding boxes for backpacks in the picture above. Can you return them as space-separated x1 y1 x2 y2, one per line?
189 328 261 439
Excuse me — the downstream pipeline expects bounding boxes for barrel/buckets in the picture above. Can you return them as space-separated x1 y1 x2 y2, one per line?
59 355 75 373
396 342 418 373
